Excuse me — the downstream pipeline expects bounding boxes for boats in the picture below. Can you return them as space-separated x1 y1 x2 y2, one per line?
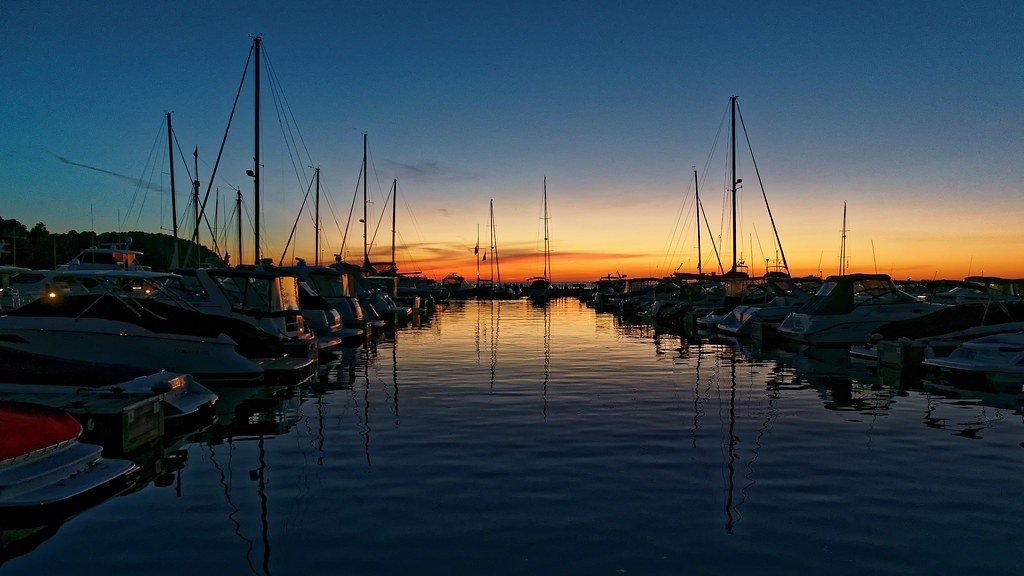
777 272 935 347
0 269 263 387
919 331 1023 382
850 309 1023 365
0 403 141 507
715 277 822 334
1 369 221 452
923 276 1024 314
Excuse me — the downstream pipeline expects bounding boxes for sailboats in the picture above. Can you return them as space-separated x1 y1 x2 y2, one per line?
12 32 781 386
